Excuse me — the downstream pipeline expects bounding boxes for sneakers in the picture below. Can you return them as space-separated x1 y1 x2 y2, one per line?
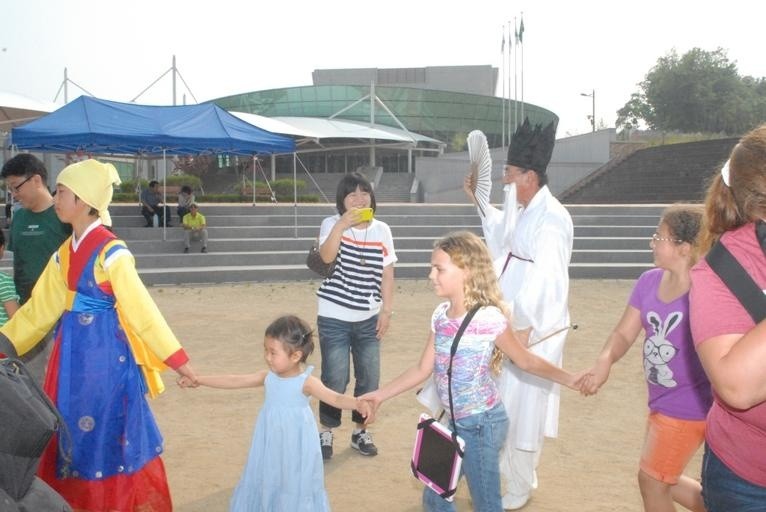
320 431 334 460
201 247 207 253
351 429 378 456
184 248 190 253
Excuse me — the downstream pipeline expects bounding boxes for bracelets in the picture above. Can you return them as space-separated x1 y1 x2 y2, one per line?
385 310 395 319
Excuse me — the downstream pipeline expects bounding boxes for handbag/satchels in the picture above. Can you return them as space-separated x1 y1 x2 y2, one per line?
306 247 335 278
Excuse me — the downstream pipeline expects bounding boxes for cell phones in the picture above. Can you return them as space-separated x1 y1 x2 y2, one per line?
352 208 374 222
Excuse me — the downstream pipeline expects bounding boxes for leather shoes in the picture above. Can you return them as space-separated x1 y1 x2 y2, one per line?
500 491 531 509
144 223 153 227
159 222 171 227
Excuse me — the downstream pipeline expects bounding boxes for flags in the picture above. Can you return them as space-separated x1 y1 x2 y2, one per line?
501 19 526 56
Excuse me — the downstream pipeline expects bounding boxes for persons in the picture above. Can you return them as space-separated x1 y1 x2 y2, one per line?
0 152 72 306
307 173 398 459
461 117 575 511
0 157 196 511
356 230 594 512
182 204 208 253
140 180 174 227
0 231 20 326
178 186 195 222
576 207 708 512
178 313 379 512
689 126 764 510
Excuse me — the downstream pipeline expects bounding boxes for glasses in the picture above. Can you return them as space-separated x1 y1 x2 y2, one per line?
6 175 33 194
653 233 684 245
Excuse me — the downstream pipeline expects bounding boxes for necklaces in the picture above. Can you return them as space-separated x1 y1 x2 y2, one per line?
346 223 369 266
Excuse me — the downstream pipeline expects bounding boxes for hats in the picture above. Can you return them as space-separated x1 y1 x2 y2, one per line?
506 118 554 172
189 201 197 207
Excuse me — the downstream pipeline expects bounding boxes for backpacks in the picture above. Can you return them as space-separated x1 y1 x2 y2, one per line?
0 331 73 501
